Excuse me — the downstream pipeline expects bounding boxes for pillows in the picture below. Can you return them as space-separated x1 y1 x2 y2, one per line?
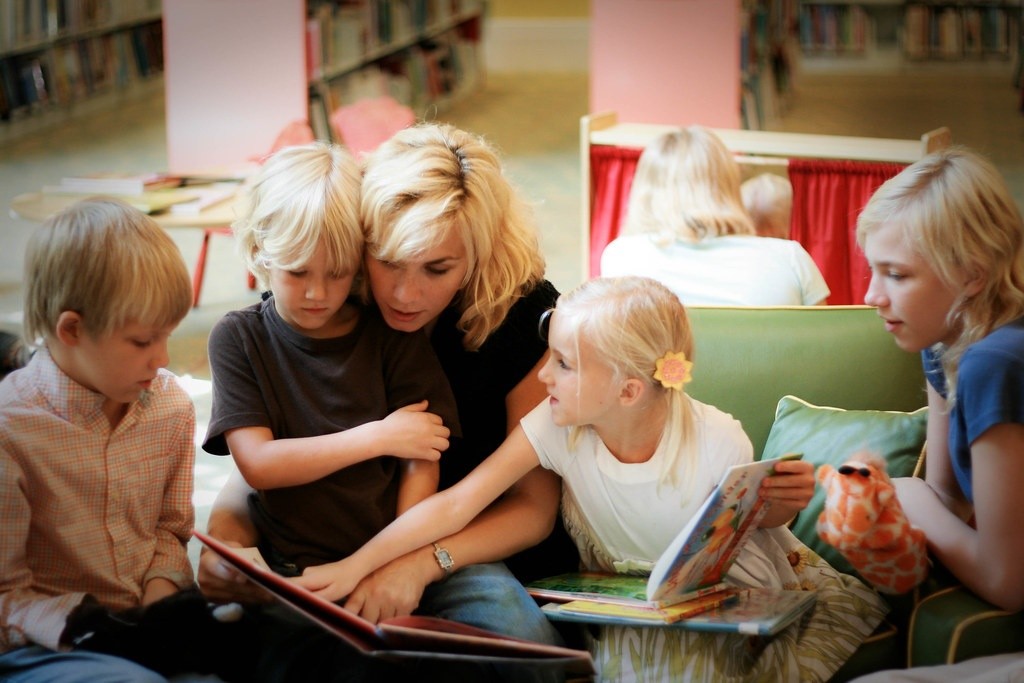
760 395 929 593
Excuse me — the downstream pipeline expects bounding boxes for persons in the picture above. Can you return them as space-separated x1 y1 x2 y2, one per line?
285 273 882 683
205 123 451 683
196 120 558 683
598 120 826 305
823 144 1024 657
1 198 219 683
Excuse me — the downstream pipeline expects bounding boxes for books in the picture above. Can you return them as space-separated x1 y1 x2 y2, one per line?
524 451 819 634
190 524 592 663
306 0 483 104
737 0 1024 131
0 0 165 117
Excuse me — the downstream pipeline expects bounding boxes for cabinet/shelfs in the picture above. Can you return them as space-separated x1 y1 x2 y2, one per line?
587 0 798 130
0 0 164 148
161 0 486 178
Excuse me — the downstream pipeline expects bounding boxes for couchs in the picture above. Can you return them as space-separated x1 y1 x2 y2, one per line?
684 303 1024 683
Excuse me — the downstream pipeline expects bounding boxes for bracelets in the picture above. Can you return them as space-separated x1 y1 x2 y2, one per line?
433 543 454 576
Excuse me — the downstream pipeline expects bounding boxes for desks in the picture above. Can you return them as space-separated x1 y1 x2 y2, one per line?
8 169 258 309
579 106 951 305
